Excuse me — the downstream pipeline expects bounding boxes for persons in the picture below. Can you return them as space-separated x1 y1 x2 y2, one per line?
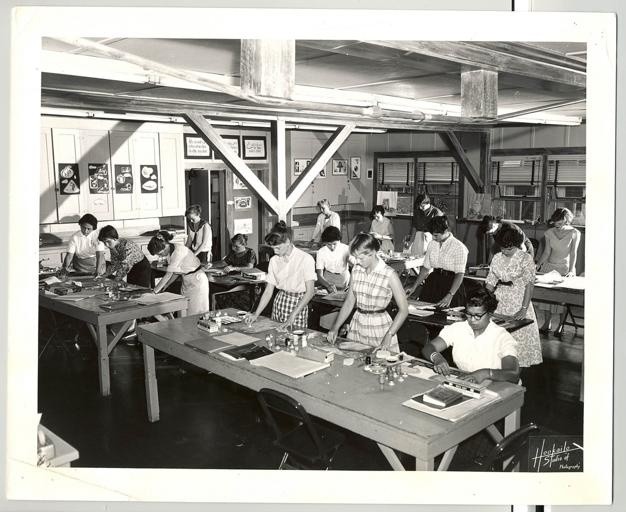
147 231 210 316
204 232 257 272
369 205 395 252
62 213 105 280
244 220 318 332
311 198 340 243
480 215 535 268
404 194 443 255
404 216 469 309
93 225 150 339
421 286 520 384
326 233 409 355
184 205 213 262
535 207 581 336
316 225 354 293
485 224 544 367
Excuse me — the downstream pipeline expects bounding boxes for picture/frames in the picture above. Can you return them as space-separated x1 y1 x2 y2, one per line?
350 156 361 179
242 135 267 160
215 135 240 158
332 159 348 177
294 157 313 177
183 132 212 160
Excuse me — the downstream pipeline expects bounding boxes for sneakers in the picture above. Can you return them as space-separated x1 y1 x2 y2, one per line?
123 330 136 338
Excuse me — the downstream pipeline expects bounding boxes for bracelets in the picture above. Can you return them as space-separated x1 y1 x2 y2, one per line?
488 369 494 380
521 306 527 309
385 332 395 337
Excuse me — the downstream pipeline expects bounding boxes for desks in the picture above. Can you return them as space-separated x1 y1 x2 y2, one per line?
263 241 406 270
40 270 190 397
148 257 267 311
462 261 585 344
311 283 535 366
138 307 525 471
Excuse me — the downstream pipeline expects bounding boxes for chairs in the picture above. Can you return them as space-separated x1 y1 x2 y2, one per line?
257 388 349 469
38 305 73 360
396 324 430 361
486 423 543 472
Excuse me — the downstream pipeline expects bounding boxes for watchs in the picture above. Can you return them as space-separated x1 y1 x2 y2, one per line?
430 351 441 362
447 291 455 296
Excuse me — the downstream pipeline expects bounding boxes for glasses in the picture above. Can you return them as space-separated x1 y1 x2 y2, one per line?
464 311 488 320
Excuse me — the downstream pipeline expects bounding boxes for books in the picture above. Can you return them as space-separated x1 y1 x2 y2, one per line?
422 386 464 407
